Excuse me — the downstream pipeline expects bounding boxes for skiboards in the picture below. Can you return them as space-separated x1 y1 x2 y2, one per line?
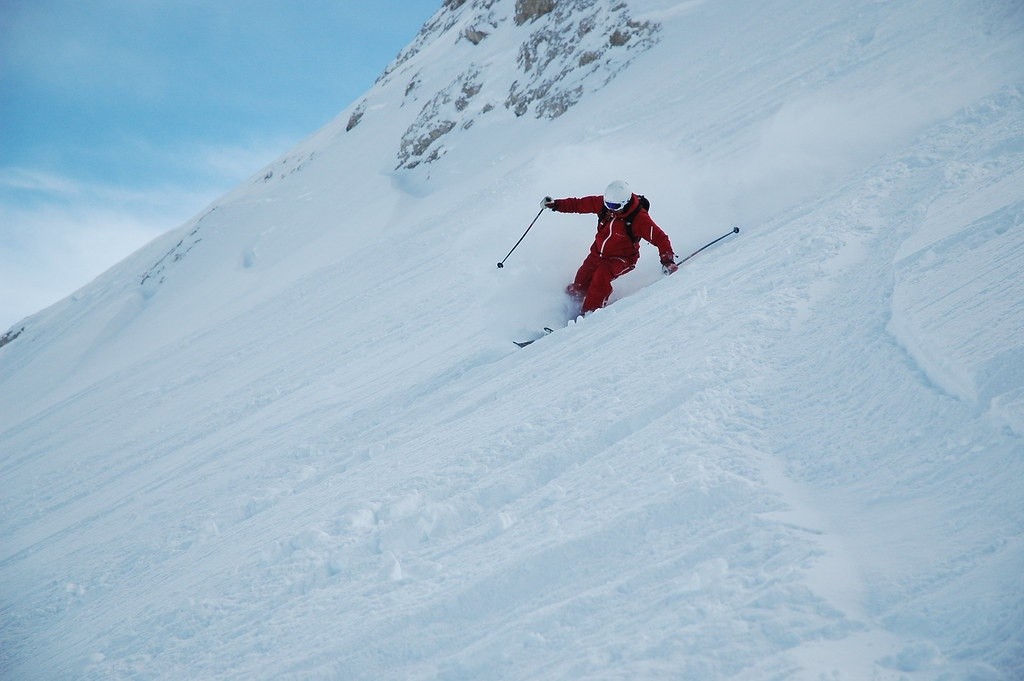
512 311 591 348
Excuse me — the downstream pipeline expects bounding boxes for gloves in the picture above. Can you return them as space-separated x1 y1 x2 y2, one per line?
660 261 679 275
539 196 554 210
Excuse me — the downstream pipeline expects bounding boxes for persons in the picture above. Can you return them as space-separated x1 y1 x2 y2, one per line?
540 181 678 316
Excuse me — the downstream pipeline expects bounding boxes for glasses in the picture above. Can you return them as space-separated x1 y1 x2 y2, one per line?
604 201 623 211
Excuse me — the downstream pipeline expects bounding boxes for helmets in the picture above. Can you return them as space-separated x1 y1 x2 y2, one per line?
604 180 633 211
